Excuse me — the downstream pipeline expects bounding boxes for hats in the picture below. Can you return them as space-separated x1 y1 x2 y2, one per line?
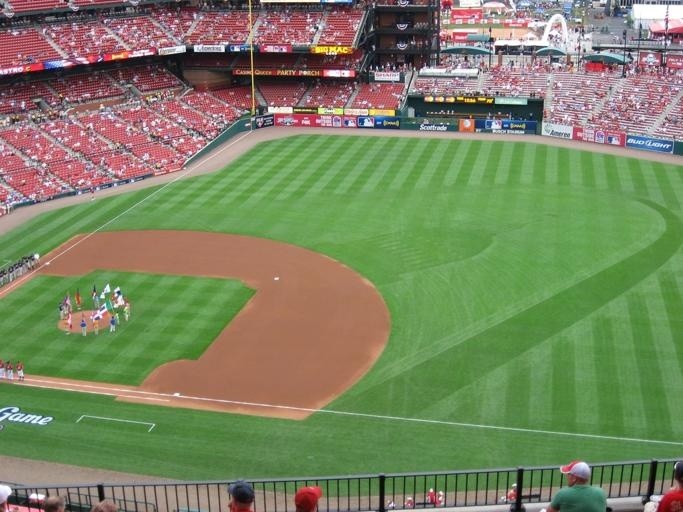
560 461 590 479
386 488 443 504
0 485 12 506
673 461 683 480
227 482 255 503
501 484 516 502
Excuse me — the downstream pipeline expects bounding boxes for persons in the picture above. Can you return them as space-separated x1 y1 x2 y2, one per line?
57 302 63 319
0 250 41 288
545 460 608 511
654 460 682 511
15 359 25 382
0 359 5 380
382 487 445 509
497 483 517 503
4 360 14 382
109 315 115 332
41 494 65 511
92 318 99 335
62 317 70 335
225 480 256 512
79 319 86 337
0 484 13 512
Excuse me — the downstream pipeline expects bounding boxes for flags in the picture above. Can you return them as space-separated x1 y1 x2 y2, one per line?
61 282 131 329
